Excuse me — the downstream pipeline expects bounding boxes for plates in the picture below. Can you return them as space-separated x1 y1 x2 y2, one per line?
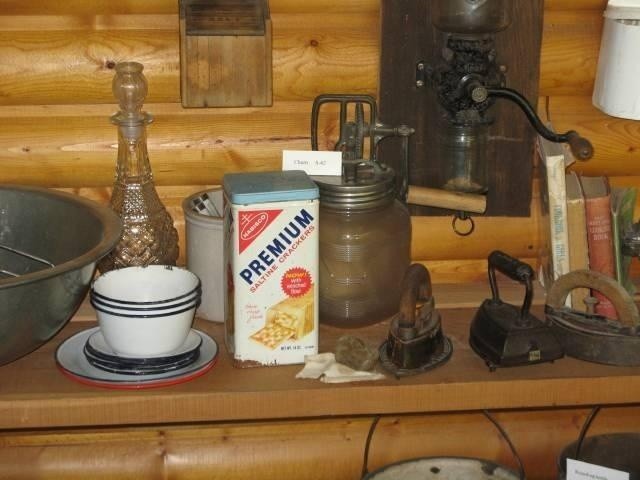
55 326 219 390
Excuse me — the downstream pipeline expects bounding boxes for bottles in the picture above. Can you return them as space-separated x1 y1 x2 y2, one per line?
308 93 489 330
96 60 181 284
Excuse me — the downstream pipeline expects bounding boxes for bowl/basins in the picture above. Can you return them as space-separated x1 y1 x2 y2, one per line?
0 181 125 370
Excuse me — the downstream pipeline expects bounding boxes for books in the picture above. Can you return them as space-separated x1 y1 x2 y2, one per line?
539 121 639 323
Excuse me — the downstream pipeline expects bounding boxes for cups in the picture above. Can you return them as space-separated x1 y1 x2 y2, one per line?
89 265 202 356
